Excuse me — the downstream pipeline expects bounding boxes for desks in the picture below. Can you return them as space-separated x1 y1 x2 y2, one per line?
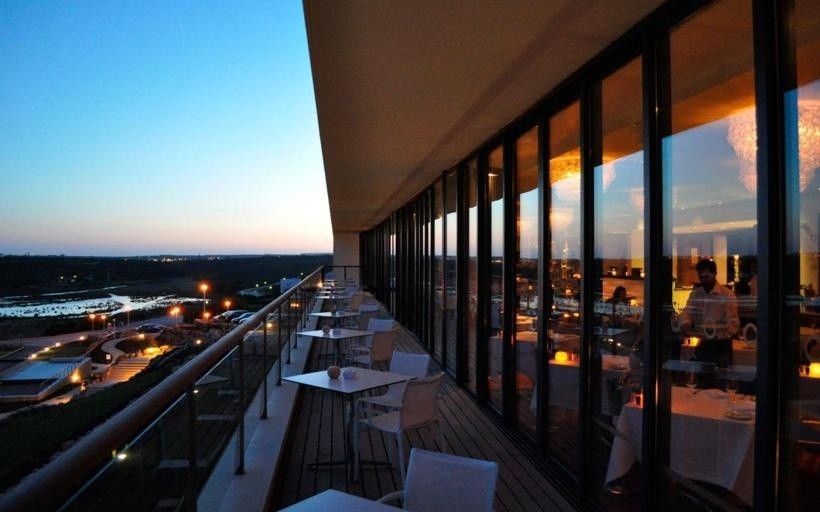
277 278 416 510
491 314 757 506
489 315 756 487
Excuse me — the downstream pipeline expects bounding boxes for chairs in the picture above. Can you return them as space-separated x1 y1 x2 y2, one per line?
349 351 503 512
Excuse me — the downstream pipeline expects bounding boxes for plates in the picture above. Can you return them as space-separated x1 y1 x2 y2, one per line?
698 323 733 330
724 411 753 420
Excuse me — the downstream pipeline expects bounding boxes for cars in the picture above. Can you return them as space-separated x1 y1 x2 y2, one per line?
213 308 261 330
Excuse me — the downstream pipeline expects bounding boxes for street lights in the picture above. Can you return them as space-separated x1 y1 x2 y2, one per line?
89 313 108 333
169 282 231 346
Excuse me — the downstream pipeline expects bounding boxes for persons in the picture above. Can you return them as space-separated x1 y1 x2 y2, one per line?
805 282 817 301
603 285 631 305
622 264 629 277
627 257 741 391
